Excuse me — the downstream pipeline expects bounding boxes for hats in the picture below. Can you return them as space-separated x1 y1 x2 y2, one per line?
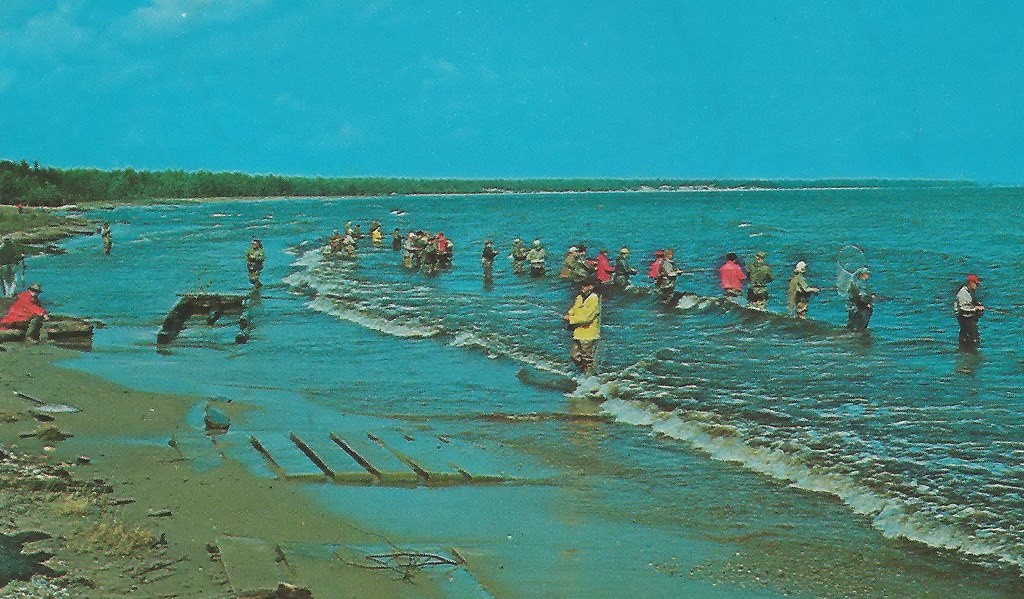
534 240 540 246
333 230 339 234
967 273 980 283
514 239 520 242
426 235 433 240
347 229 353 234
621 248 628 254
569 246 577 252
348 221 353 227
252 235 261 243
580 244 587 250
582 276 595 283
656 250 663 256
599 249 608 254
859 267 870 273
756 250 764 257
484 240 492 244
28 282 43 293
796 262 807 269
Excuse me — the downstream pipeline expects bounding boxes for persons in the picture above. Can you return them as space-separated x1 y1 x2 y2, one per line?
846 268 877 328
564 276 601 371
0 283 49 345
482 240 499 271
512 239 547 273
560 246 598 277
789 261 819 318
0 236 25 297
747 253 773 308
246 238 265 286
954 275 983 342
370 221 382 247
393 229 455 269
322 222 362 253
719 253 745 298
596 247 638 285
100 221 113 252
649 250 682 289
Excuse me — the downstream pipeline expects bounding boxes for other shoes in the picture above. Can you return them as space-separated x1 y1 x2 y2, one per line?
26 337 39 343
